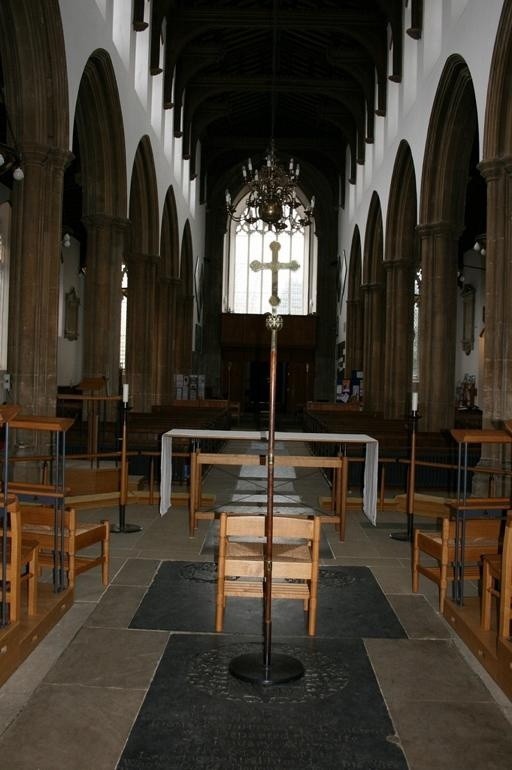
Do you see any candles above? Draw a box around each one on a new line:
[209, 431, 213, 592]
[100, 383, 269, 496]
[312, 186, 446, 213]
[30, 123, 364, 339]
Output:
[412, 392, 418, 411]
[122, 383, 129, 403]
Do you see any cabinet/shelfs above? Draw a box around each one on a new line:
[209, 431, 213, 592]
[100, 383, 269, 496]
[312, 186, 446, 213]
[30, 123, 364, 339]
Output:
[449, 428, 512, 609]
[0, 402, 23, 630]
[0, 416, 75, 593]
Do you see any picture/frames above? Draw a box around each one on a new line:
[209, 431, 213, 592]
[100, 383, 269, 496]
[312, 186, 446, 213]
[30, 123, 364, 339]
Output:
[64, 287, 80, 341]
[195, 324, 201, 353]
[459, 283, 476, 356]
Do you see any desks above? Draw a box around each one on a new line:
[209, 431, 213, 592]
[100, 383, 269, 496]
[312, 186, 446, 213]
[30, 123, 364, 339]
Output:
[159, 428, 380, 545]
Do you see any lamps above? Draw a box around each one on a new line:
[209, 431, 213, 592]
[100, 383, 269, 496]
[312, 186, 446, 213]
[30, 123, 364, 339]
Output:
[473, 233, 486, 255]
[457, 271, 465, 282]
[223, 137, 317, 232]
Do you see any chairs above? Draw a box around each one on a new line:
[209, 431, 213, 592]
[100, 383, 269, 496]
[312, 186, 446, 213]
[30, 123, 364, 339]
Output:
[214, 512, 321, 636]
[0, 492, 40, 626]
[480, 516, 512, 640]
[0, 505, 111, 589]
[410, 517, 507, 616]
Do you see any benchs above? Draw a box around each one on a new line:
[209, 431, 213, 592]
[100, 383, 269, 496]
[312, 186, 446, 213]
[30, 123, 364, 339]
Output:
[56, 406, 230, 480]
[301, 409, 481, 486]
